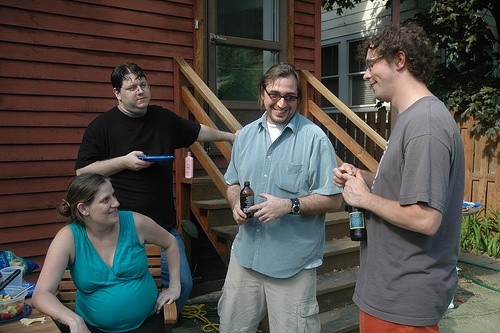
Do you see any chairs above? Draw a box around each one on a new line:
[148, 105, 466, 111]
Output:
[58, 244, 177, 333]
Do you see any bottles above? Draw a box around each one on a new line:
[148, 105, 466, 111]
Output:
[184, 152, 193, 178]
[240, 181, 254, 219]
[347, 205, 368, 242]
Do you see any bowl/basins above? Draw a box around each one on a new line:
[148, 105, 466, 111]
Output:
[0, 286, 28, 320]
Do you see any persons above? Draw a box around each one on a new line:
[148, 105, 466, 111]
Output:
[70, 62, 244, 321]
[30, 171, 182, 333]
[216, 67, 348, 333]
[332, 26, 465, 333]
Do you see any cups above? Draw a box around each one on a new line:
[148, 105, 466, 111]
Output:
[0, 266, 24, 287]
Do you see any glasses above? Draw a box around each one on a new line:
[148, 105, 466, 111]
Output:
[263, 86, 299, 102]
[366, 55, 385, 72]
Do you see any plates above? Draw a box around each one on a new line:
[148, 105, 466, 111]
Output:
[0, 282, 36, 296]
[0, 302, 31, 323]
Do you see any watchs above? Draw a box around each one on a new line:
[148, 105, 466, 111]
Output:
[290, 197, 301, 216]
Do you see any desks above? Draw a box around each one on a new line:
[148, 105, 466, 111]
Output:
[462, 205, 479, 216]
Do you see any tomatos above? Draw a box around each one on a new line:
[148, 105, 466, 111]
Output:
[3, 305, 22, 319]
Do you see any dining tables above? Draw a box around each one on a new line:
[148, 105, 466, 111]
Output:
[0, 279, 61, 333]
